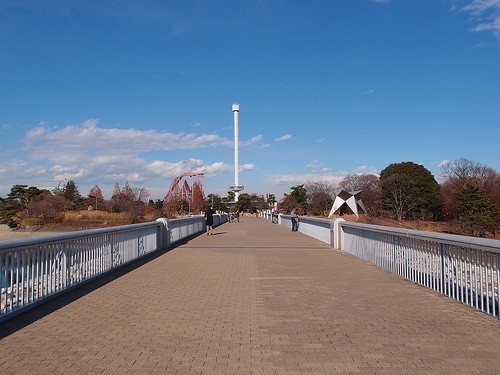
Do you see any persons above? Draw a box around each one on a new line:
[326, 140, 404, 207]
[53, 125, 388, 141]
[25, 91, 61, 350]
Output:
[7, 218, 18, 228]
[290, 208, 298, 232]
[204, 204, 215, 235]
[227, 210, 243, 223]
[251, 209, 258, 218]
